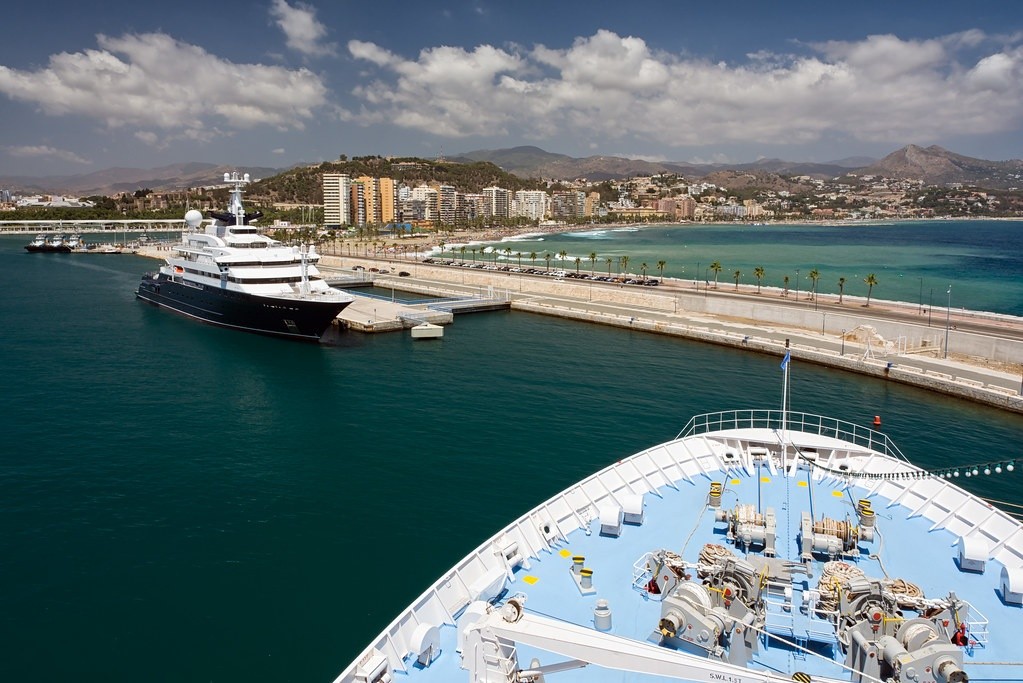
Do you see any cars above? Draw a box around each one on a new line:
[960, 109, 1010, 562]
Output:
[369, 268, 378, 272]
[379, 270, 389, 274]
[352, 265, 364, 270]
[399, 271, 410, 277]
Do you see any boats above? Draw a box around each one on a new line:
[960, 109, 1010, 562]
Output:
[134, 170, 355, 343]
[423, 258, 659, 287]
[23, 225, 50, 252]
[58, 226, 89, 253]
[41, 226, 67, 252]
